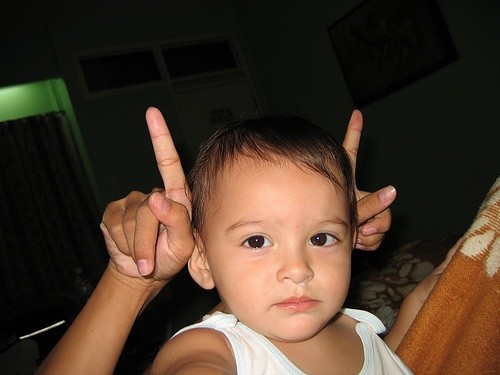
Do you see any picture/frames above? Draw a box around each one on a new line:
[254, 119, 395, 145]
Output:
[328, 0, 459, 108]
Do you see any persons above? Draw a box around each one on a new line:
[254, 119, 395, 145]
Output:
[32, 103, 397, 375]
[150, 113, 464, 375]
